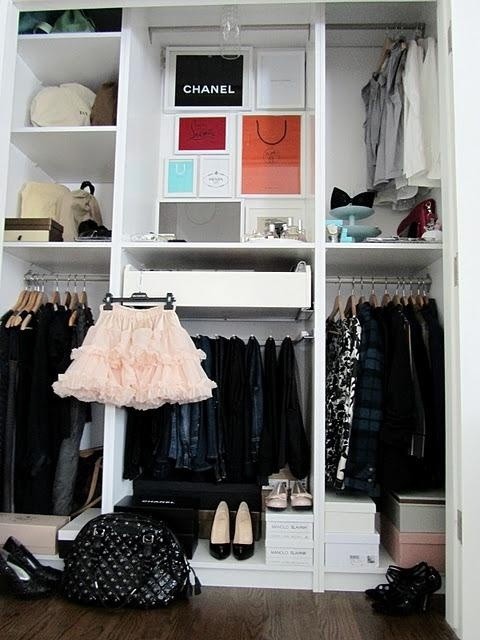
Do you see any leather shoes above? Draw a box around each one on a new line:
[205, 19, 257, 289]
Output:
[210, 500, 231, 560]
[232, 501, 254, 560]
[291, 480, 313, 509]
[0, 536, 64, 601]
[266, 481, 288, 511]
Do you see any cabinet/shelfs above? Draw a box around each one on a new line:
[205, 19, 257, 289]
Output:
[0, 0, 453, 595]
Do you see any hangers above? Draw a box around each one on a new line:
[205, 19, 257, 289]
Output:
[0, 266, 90, 330]
[369, 19, 425, 83]
[327, 274, 430, 336]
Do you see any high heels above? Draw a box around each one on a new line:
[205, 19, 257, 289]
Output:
[365, 562, 441, 611]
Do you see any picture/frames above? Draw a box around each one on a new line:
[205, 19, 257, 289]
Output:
[152, 42, 313, 243]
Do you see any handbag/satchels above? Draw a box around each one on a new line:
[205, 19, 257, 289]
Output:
[65, 511, 202, 609]
[397, 199, 436, 239]
[59, 181, 102, 242]
[72, 446, 102, 510]
[242, 115, 300, 195]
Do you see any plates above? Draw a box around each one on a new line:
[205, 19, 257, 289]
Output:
[338, 225, 382, 243]
[330, 205, 375, 220]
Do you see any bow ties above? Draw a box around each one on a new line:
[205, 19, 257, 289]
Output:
[329, 186, 376, 210]
